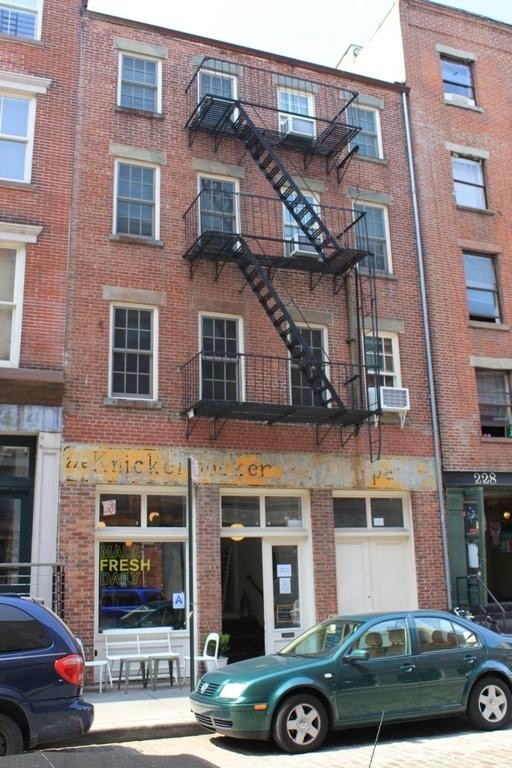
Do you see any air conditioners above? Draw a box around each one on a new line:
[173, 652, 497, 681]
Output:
[288, 234, 322, 259]
[283, 116, 317, 140]
[368, 385, 411, 413]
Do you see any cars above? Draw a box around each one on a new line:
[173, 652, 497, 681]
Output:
[189, 603, 512, 750]
[118, 599, 186, 629]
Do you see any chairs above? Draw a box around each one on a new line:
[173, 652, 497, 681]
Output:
[365, 630, 406, 659]
[419, 630, 465, 653]
[75, 626, 220, 696]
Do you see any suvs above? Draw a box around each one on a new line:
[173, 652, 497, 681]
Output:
[0, 589, 96, 754]
[98, 586, 165, 631]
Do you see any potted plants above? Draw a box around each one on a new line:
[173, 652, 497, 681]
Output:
[201, 631, 230, 673]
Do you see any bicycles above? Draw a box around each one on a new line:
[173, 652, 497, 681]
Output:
[451, 605, 496, 633]
